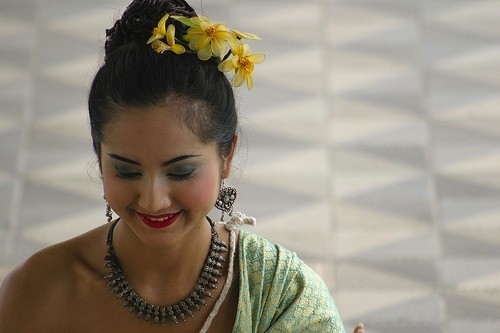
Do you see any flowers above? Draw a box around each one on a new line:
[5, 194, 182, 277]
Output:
[147, 13, 266, 91]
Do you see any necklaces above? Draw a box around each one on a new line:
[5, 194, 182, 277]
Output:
[101, 215, 229, 327]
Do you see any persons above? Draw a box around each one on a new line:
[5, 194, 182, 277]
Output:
[0, 0, 365, 333]
